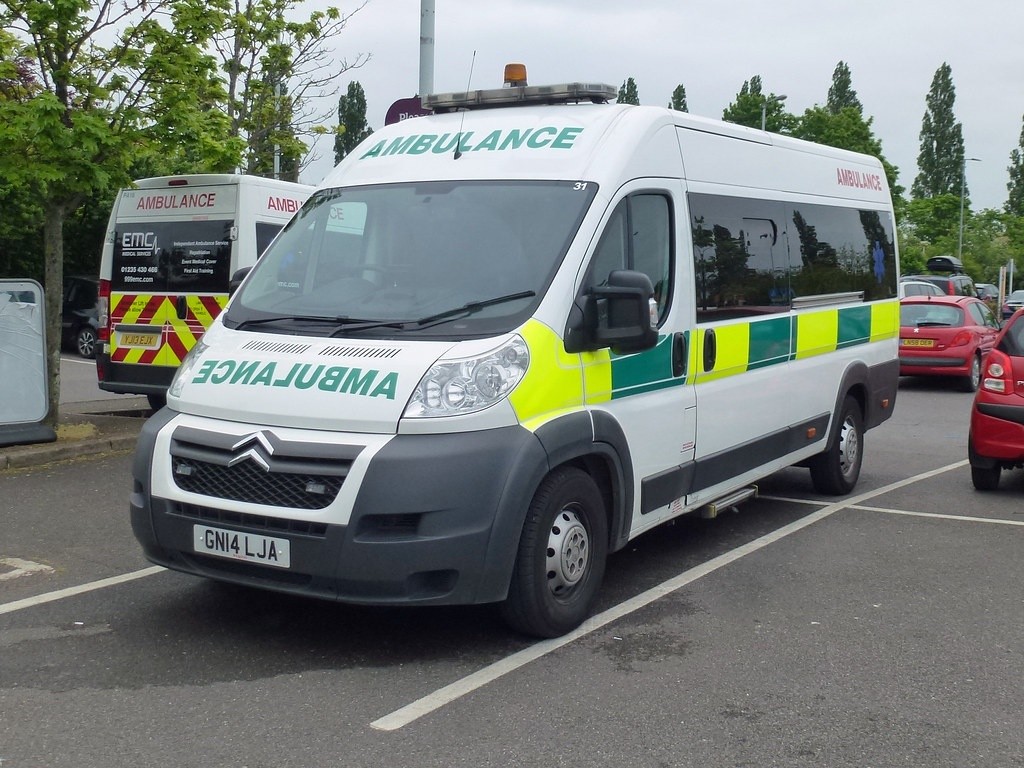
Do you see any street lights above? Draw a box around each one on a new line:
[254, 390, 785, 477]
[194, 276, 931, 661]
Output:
[958, 157, 982, 260]
[759, 94, 788, 131]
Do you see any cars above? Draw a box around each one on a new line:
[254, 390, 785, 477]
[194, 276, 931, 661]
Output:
[974, 282, 999, 300]
[900, 295, 1002, 392]
[967, 307, 1024, 492]
[1004, 290, 1024, 307]
[62, 273, 99, 359]
[899, 281, 946, 299]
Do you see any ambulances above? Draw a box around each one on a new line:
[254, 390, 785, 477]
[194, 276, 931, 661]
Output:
[95, 171, 367, 403]
[127, 64, 899, 638]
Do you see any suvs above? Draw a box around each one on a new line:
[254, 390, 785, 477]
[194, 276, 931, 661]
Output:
[900, 254, 979, 297]
